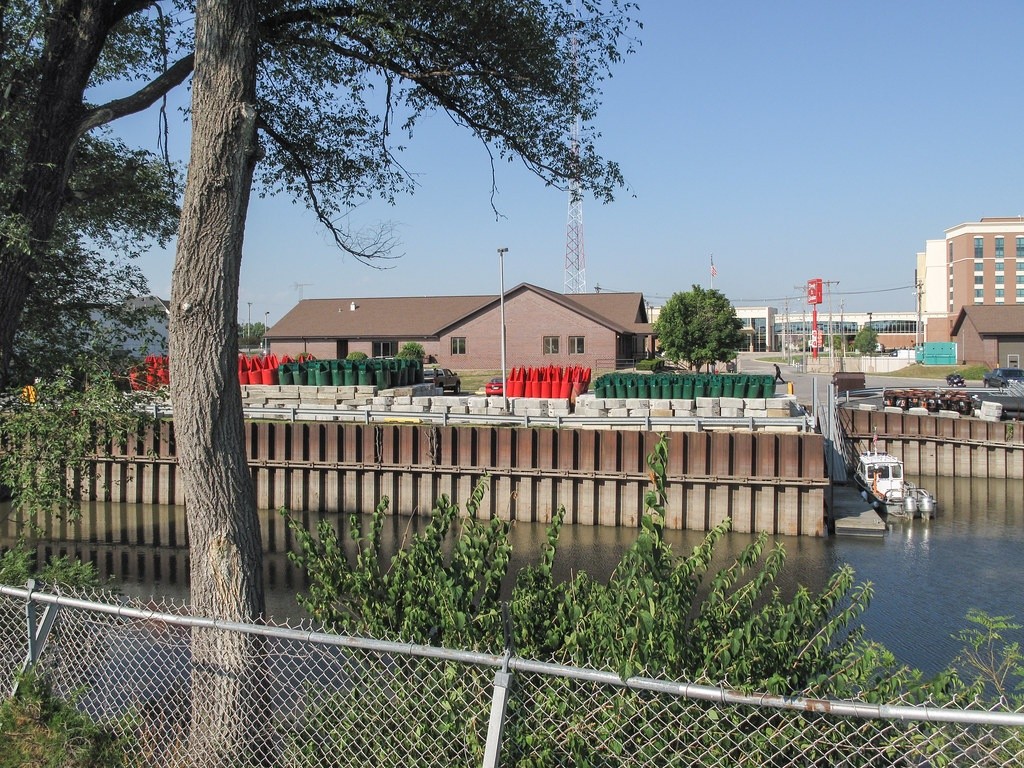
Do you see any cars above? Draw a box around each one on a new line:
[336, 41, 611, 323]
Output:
[889, 350, 898, 357]
[485, 376, 508, 397]
[875, 343, 886, 354]
[983, 367, 1024, 390]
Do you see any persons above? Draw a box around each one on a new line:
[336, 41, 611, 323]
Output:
[774, 364, 785, 384]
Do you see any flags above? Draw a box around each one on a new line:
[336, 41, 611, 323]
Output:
[711, 253, 717, 289]
[873, 427, 878, 455]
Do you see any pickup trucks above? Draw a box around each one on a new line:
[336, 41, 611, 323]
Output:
[423, 368, 461, 394]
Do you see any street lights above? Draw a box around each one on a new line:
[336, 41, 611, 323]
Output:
[248, 303, 253, 344]
[867, 312, 873, 358]
[496, 247, 511, 416]
[264, 312, 270, 354]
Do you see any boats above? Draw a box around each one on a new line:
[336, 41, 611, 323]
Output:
[855, 427, 938, 522]
